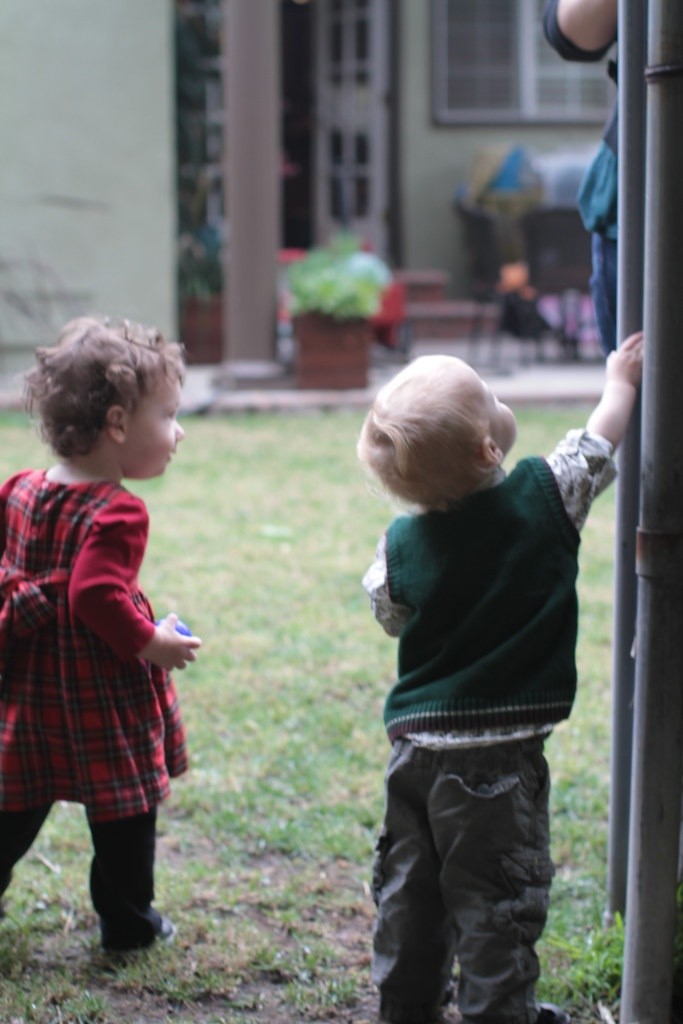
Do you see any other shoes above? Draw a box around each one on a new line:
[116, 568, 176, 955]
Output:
[534, 1004, 570, 1024]
[103, 917, 176, 953]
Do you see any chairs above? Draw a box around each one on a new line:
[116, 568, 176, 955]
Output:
[452, 200, 592, 367]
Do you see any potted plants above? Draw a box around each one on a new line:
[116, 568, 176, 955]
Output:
[282, 235, 394, 390]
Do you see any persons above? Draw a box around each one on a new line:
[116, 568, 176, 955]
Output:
[544, 0, 618, 355]
[355, 328, 645, 1024]
[0, 318, 201, 951]
